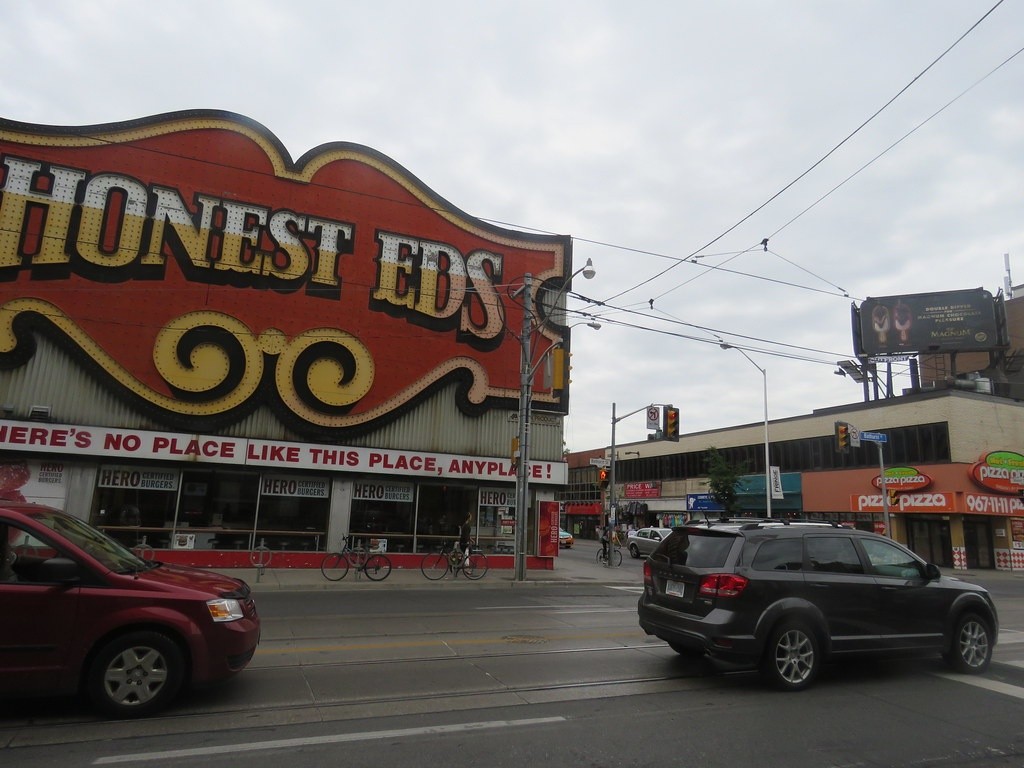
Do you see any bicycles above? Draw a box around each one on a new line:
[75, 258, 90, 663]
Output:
[596, 542, 624, 567]
[420, 537, 489, 581]
[321, 533, 392, 581]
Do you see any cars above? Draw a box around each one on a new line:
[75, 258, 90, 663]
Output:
[626, 528, 673, 559]
[558, 526, 575, 548]
[1, 498, 260, 720]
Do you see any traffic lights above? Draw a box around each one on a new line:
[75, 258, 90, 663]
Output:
[662, 406, 680, 442]
[834, 420, 852, 454]
[599, 469, 609, 485]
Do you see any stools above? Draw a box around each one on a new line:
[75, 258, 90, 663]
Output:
[257, 542, 268, 550]
[159, 538, 170, 549]
[436, 545, 443, 553]
[300, 542, 310, 551]
[208, 539, 219, 548]
[277, 541, 289, 551]
[233, 540, 244, 550]
[396, 544, 404, 552]
[417, 545, 424, 552]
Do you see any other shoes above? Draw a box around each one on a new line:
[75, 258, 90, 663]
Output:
[449, 564, 453, 573]
[603, 560, 607, 563]
[464, 570, 472, 575]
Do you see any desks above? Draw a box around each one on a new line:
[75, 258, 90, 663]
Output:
[94, 525, 516, 555]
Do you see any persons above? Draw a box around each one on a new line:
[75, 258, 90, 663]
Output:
[460, 513, 475, 575]
[0, 539, 19, 582]
[601, 526, 622, 565]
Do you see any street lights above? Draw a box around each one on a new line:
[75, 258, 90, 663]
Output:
[718, 341, 773, 520]
[512, 260, 602, 580]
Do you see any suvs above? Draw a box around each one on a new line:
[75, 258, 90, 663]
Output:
[636, 517, 1000, 693]
